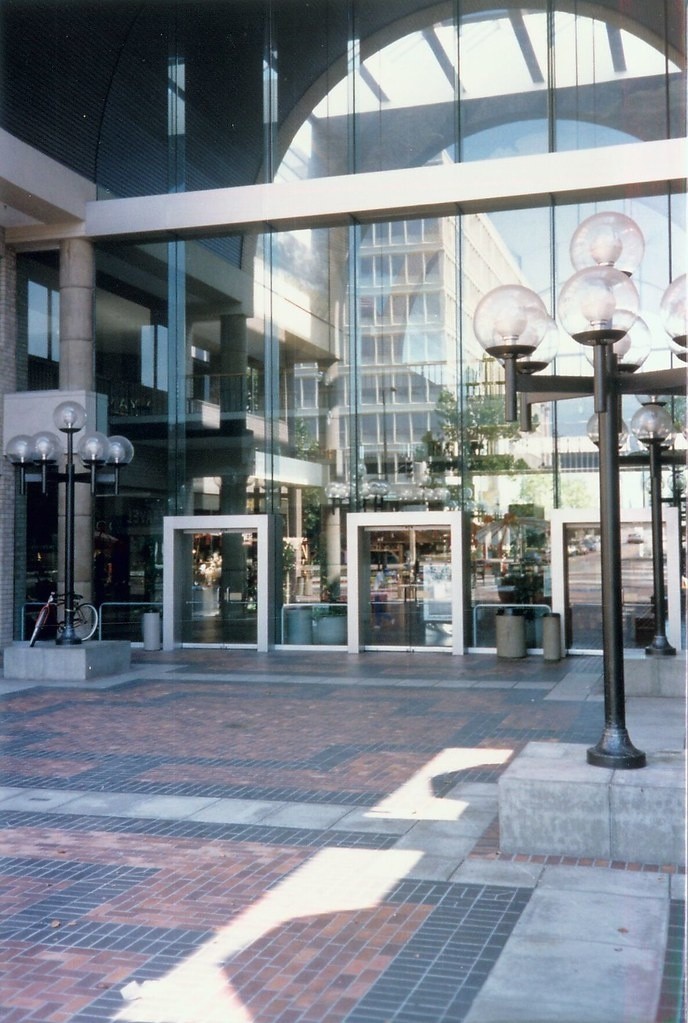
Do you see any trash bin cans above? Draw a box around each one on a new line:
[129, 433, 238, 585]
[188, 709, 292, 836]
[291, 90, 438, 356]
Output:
[143, 608, 162, 652]
[496, 606, 528, 659]
[541, 612, 561, 661]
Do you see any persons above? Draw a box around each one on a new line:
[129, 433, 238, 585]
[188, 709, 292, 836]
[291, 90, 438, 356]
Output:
[500, 552, 507, 576]
[373, 568, 396, 630]
[93, 544, 112, 590]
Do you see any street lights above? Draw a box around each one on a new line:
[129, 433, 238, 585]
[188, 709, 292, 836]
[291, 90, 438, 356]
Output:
[585, 391, 678, 656]
[472, 211, 687, 769]
[6, 400, 136, 647]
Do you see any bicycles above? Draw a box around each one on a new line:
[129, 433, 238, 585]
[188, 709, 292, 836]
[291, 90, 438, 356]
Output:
[28, 577, 99, 646]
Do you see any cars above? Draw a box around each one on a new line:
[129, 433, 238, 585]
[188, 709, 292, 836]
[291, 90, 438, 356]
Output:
[322, 532, 644, 615]
